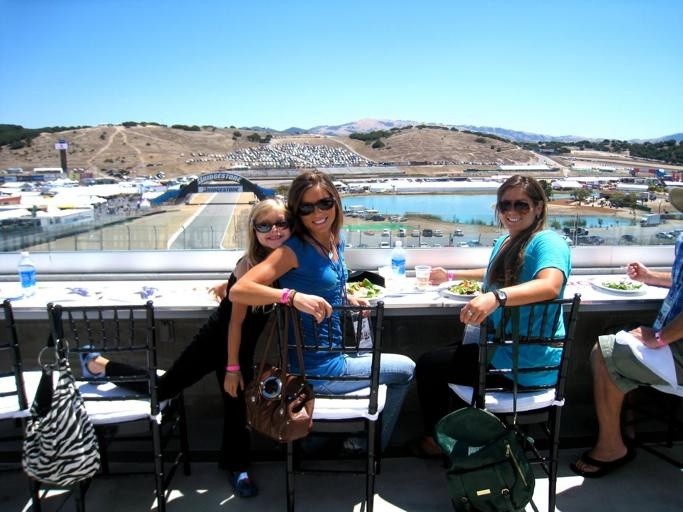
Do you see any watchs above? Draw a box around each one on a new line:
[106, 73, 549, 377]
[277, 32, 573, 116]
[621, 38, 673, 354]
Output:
[492, 289, 507, 306]
[286, 288, 296, 308]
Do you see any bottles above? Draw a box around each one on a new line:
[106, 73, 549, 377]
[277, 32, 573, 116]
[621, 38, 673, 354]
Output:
[18, 252, 36, 297]
[392, 241, 406, 279]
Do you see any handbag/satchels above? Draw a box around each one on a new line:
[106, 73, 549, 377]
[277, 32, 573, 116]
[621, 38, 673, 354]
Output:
[244, 365, 314, 444]
[430, 405, 534, 511]
[20, 359, 101, 487]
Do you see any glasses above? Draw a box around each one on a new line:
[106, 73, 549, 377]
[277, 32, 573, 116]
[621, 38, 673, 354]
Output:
[497, 201, 530, 215]
[253, 220, 289, 233]
[297, 197, 336, 216]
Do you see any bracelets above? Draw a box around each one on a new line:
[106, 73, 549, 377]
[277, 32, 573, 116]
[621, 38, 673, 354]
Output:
[447, 271, 455, 281]
[224, 366, 241, 371]
[281, 287, 289, 304]
[654, 330, 667, 347]
[225, 372, 242, 376]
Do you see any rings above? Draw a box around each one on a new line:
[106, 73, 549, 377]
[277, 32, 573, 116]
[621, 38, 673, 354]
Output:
[467, 309, 474, 318]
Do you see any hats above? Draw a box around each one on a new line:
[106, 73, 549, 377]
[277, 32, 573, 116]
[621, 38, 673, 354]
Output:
[670, 188, 683, 213]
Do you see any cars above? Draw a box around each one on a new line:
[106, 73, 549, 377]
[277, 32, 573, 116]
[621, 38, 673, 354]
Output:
[543, 168, 682, 202]
[562, 213, 683, 245]
[342, 209, 499, 247]
[1, 167, 199, 215]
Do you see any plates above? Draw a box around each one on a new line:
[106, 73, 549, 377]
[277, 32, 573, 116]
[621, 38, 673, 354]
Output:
[589, 275, 648, 294]
[439, 280, 483, 297]
[346, 281, 386, 301]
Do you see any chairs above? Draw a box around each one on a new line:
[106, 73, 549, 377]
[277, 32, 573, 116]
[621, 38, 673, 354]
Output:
[272, 300, 388, 512]
[634, 385, 683, 469]
[448, 293, 580, 509]
[0, 300, 47, 512]
[45, 301, 188, 511]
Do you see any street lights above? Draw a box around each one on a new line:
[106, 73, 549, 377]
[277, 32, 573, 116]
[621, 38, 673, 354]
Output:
[659, 199, 670, 213]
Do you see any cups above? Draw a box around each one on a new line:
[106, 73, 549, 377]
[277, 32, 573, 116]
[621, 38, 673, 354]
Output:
[415, 265, 431, 289]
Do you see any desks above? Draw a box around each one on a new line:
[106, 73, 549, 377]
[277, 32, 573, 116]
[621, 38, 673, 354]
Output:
[0, 274, 670, 322]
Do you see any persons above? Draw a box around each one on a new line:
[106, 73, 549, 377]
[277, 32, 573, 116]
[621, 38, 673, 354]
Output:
[568, 187, 683, 478]
[228, 169, 417, 460]
[78, 197, 292, 497]
[414, 174, 573, 469]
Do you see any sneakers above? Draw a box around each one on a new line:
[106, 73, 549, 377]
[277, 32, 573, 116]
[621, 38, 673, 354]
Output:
[78, 344, 104, 379]
[226, 470, 259, 498]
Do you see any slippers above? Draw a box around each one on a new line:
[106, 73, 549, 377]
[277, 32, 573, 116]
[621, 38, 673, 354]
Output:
[570, 445, 629, 478]
